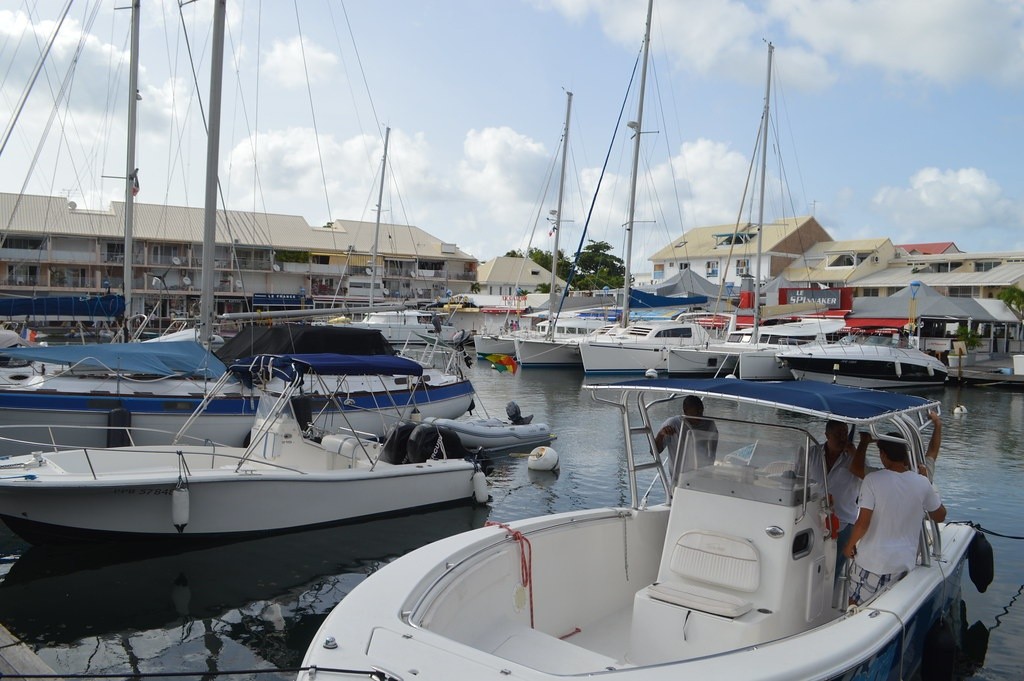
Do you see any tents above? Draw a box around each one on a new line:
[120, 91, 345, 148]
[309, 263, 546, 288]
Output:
[637, 268, 727, 299]
[845, 281, 1018, 355]
[760, 275, 799, 295]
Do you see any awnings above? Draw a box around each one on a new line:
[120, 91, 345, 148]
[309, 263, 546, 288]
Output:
[522, 309, 686, 320]
[696, 316, 755, 327]
[782, 310, 850, 319]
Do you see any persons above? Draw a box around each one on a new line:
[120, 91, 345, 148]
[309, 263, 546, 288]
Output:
[904, 331, 909, 339]
[800, 420, 870, 578]
[650, 395, 719, 498]
[135, 312, 141, 328]
[510, 320, 517, 332]
[40, 340, 47, 348]
[122, 322, 129, 344]
[851, 410, 941, 485]
[981, 327, 985, 336]
[0, 321, 4, 331]
[149, 314, 156, 329]
[17, 316, 37, 349]
[843, 431, 946, 606]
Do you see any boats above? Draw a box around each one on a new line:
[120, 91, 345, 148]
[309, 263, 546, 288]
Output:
[295, 370, 994, 681]
[1, 350, 493, 541]
[395, 403, 555, 454]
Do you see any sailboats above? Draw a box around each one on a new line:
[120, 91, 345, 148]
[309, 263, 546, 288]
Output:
[1, 0, 1024, 458]
